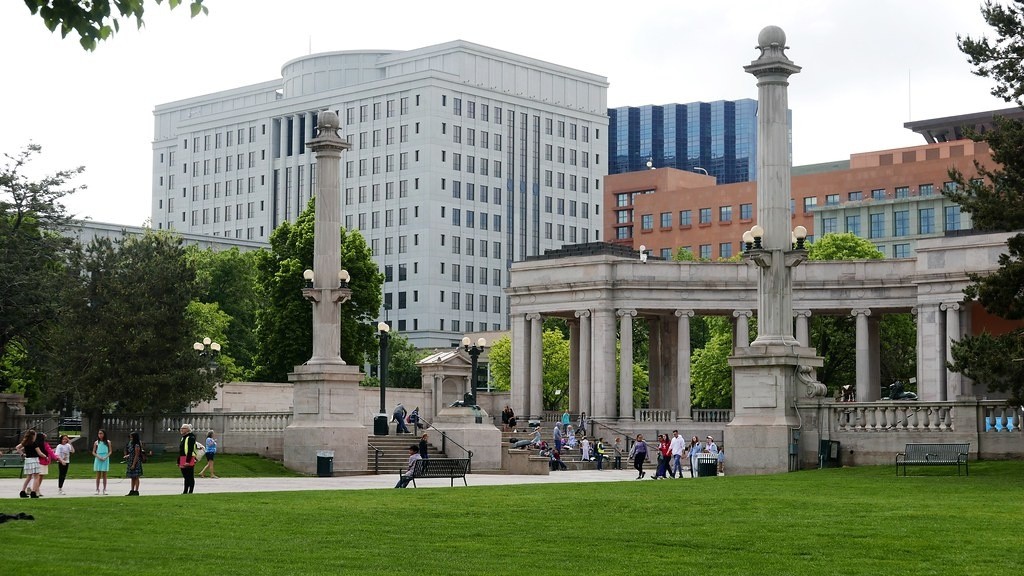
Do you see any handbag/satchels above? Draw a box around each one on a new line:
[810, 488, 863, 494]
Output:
[407, 414, 411, 423]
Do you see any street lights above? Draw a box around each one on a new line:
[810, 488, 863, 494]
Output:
[300, 109, 353, 364]
[374, 322, 391, 414]
[740, 24, 809, 347]
[461, 337, 486, 406]
[193, 337, 222, 382]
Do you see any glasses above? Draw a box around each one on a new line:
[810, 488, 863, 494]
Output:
[181, 428, 188, 430]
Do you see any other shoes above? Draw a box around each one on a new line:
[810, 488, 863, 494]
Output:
[95, 489, 100, 495]
[636, 476, 642, 479]
[651, 472, 683, 479]
[30, 491, 39, 498]
[125, 490, 134, 496]
[132, 491, 139, 496]
[26, 487, 32, 495]
[197, 473, 204, 478]
[35, 490, 43, 496]
[20, 491, 29, 498]
[211, 475, 219, 479]
[103, 489, 108, 495]
[642, 472, 645, 478]
[58, 488, 64, 495]
[404, 430, 411, 433]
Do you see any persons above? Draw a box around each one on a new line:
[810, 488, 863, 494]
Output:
[93, 429, 112, 495]
[15, 429, 75, 498]
[176, 424, 206, 494]
[501, 404, 625, 471]
[390, 403, 424, 434]
[123, 432, 143, 496]
[395, 445, 422, 488]
[419, 434, 429, 459]
[627, 430, 724, 480]
[198, 429, 218, 478]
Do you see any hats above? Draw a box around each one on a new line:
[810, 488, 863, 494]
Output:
[706, 435, 713, 440]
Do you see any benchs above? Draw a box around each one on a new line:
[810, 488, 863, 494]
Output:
[400, 457, 471, 488]
[0, 453, 25, 478]
[142, 444, 180, 463]
[895, 442, 970, 477]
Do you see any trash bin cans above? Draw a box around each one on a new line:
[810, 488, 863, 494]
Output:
[696, 452, 718, 477]
[315, 450, 334, 477]
